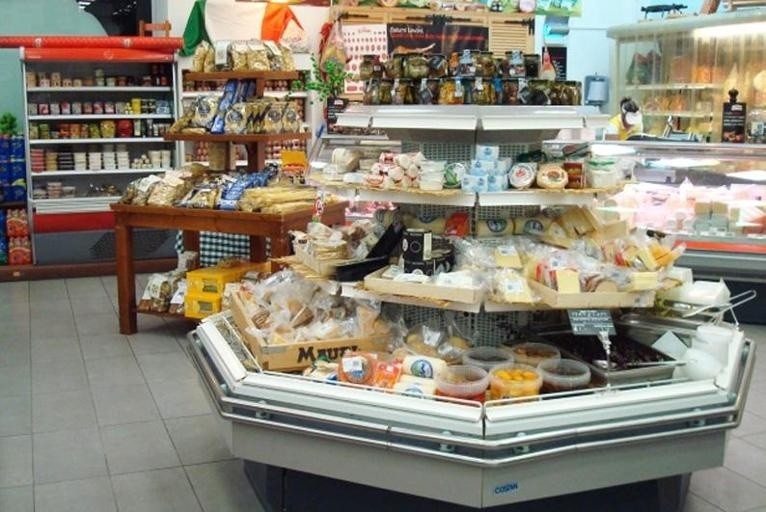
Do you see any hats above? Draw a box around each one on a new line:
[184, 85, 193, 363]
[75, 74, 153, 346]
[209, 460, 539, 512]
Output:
[622, 104, 642, 125]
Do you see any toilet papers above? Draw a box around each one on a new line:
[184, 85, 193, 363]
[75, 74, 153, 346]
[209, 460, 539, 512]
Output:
[589, 81, 607, 102]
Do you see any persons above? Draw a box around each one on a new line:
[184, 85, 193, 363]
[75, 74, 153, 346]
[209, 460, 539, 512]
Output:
[602, 96, 643, 141]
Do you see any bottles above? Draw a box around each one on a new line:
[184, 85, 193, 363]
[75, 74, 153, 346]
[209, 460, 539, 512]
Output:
[0, 209, 32, 266]
[0, 133, 27, 202]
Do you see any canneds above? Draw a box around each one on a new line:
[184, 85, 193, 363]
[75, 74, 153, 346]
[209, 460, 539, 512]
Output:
[359, 49, 583, 106]
